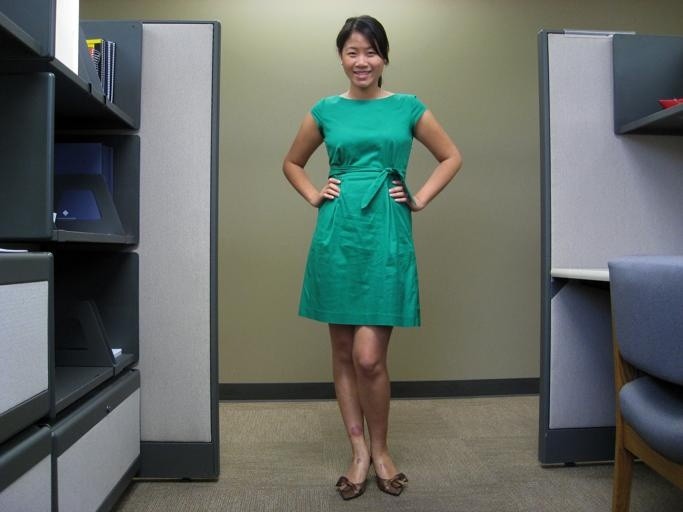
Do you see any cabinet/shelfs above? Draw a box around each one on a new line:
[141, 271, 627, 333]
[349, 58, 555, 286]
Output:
[0, 0, 141, 512]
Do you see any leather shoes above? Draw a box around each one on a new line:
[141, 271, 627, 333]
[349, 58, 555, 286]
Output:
[335, 476, 366, 500]
[375, 473, 408, 497]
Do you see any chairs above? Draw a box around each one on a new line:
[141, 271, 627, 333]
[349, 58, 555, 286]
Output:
[608, 256, 683, 512]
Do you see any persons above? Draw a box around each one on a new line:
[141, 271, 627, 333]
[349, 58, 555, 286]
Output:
[280, 13, 462, 499]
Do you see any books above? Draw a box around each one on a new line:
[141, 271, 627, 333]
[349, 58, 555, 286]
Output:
[85, 37, 115, 103]
